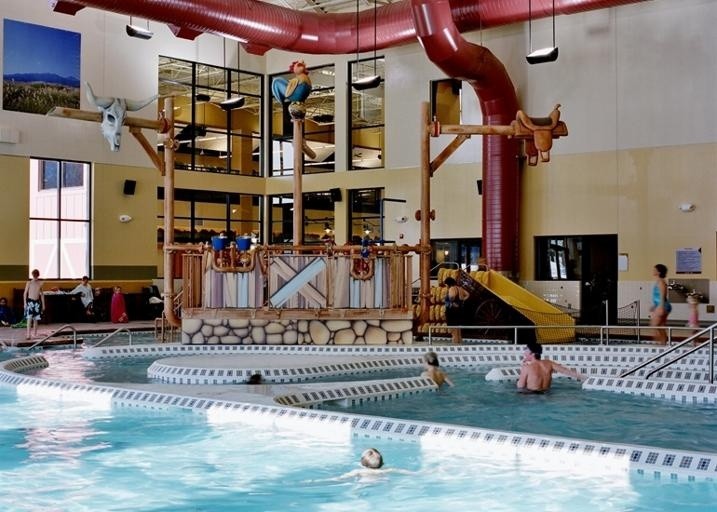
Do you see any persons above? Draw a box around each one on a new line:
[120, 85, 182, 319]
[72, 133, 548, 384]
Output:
[422, 353, 454, 390]
[42, 286, 63, 297]
[0, 297, 13, 327]
[21, 270, 44, 340]
[687, 290, 697, 342]
[444, 276, 467, 343]
[518, 342, 588, 392]
[68, 276, 102, 315]
[112, 287, 128, 323]
[647, 264, 670, 342]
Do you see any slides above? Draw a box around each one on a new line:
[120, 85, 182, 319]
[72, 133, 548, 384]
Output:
[470, 269, 577, 345]
